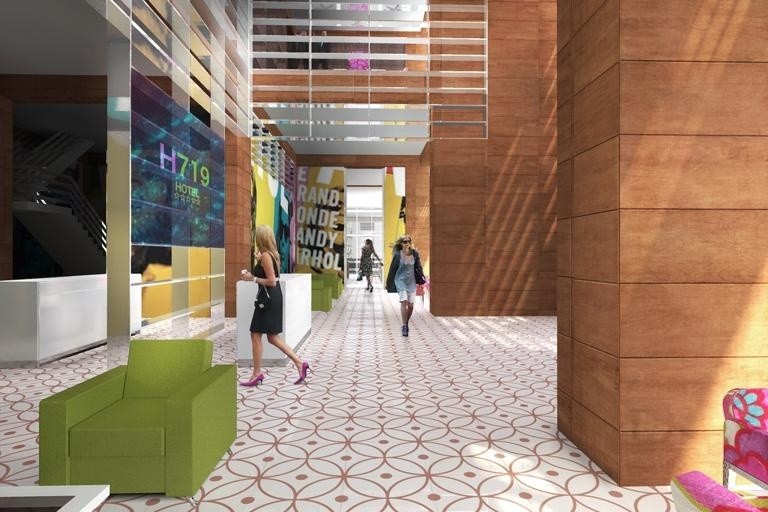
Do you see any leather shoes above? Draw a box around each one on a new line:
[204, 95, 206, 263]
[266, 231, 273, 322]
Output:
[403, 325, 408, 336]
[366, 285, 374, 293]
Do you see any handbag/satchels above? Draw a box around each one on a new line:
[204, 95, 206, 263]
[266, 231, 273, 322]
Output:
[253, 285, 272, 313]
[357, 272, 363, 281]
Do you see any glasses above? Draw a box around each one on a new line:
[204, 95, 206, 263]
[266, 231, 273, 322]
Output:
[402, 240, 411, 243]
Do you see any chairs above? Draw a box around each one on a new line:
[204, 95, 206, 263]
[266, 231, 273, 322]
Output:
[35, 338, 239, 504]
[300, 264, 344, 312]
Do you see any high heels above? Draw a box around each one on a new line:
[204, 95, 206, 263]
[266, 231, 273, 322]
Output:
[293, 362, 313, 385]
[240, 373, 264, 387]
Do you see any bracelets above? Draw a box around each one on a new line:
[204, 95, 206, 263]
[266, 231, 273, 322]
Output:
[253, 277, 257, 283]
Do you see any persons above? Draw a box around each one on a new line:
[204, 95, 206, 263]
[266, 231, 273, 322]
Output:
[385, 235, 425, 337]
[239, 225, 316, 385]
[296, 31, 310, 68]
[311, 30, 331, 69]
[358, 239, 383, 292]
